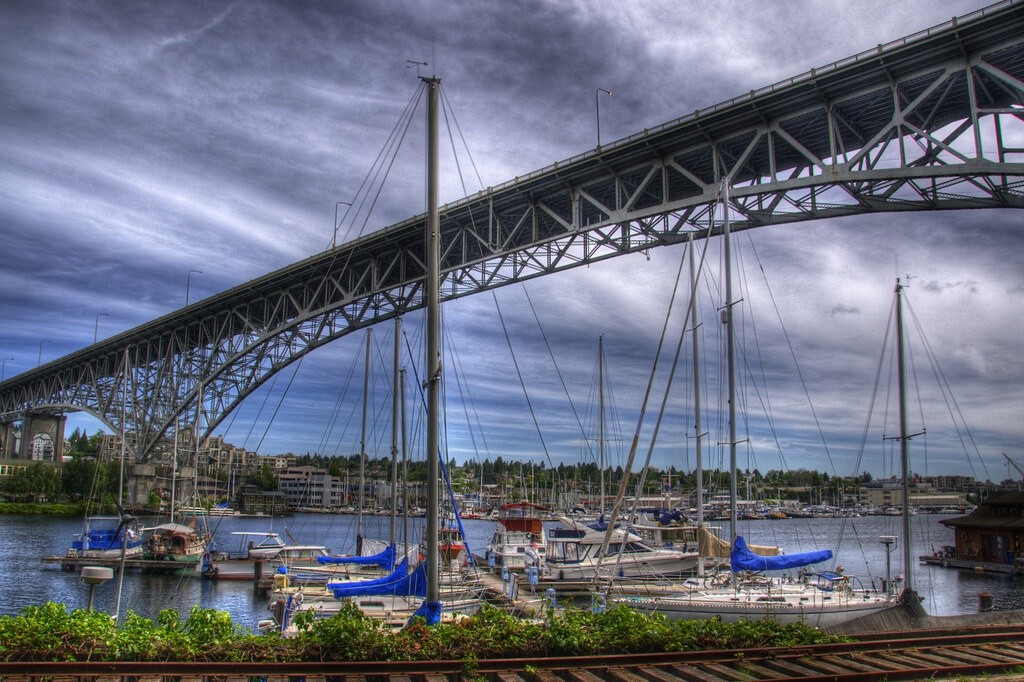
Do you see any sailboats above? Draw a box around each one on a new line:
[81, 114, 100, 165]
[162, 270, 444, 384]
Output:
[0, 175, 995, 635]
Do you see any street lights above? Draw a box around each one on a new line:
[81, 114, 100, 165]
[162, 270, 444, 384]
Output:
[185, 270, 203, 307]
[1, 357, 14, 381]
[596, 87, 614, 145]
[94, 313, 110, 343]
[38, 340, 52, 365]
[333, 202, 353, 247]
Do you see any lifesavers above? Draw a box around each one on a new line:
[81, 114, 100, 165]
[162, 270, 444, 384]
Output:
[291, 593, 304, 605]
[526, 532, 533, 539]
[960, 531, 969, 542]
